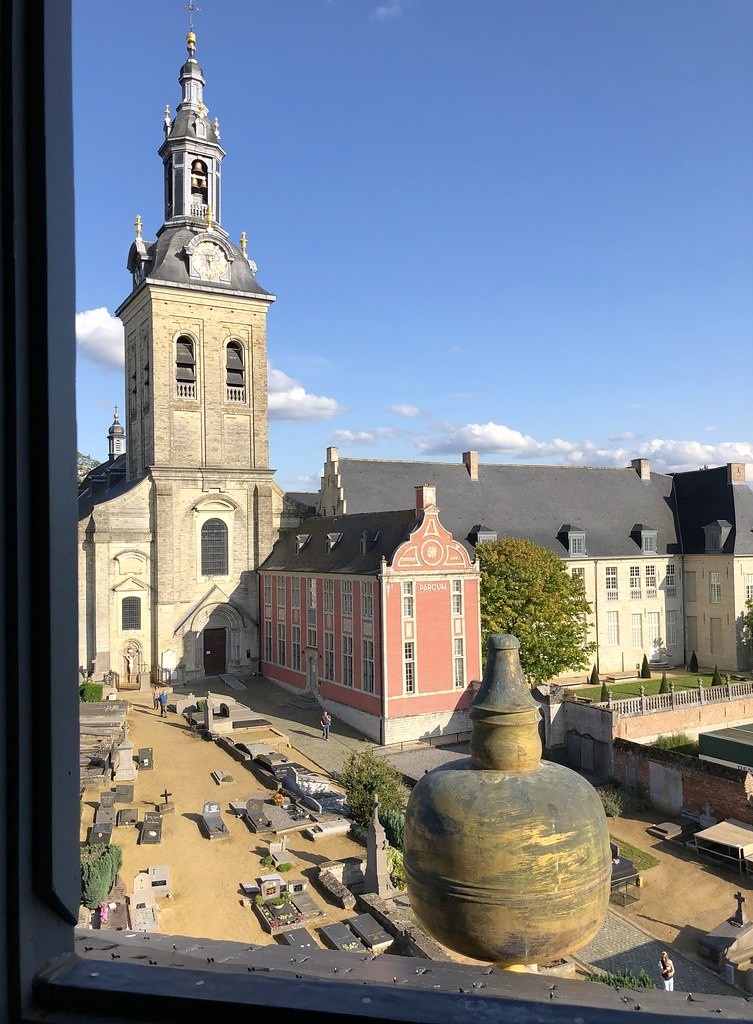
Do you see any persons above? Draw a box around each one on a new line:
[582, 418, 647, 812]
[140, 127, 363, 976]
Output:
[658, 952, 675, 991]
[126, 653, 138, 683]
[153, 686, 160, 710]
[321, 710, 331, 741]
[159, 690, 167, 718]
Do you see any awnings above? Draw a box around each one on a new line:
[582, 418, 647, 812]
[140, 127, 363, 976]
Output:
[227, 348, 245, 371]
[176, 368, 196, 381]
[227, 373, 246, 386]
[144, 376, 149, 385]
[144, 361, 149, 370]
[132, 371, 136, 378]
[132, 386, 137, 393]
[176, 344, 195, 364]
